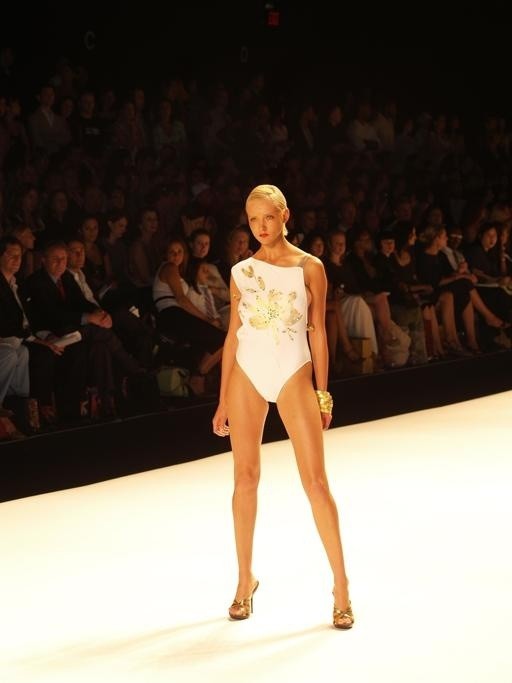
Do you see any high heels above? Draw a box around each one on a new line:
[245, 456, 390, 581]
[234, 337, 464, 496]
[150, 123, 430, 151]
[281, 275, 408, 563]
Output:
[486, 319, 511, 332]
[187, 371, 218, 402]
[467, 345, 483, 355]
[443, 338, 463, 355]
[332, 590, 354, 629]
[228, 581, 260, 619]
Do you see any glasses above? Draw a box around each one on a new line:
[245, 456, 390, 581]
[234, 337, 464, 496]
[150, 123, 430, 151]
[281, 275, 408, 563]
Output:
[450, 233, 464, 240]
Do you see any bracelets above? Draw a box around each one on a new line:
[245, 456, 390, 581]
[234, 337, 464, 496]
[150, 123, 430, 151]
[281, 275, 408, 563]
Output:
[315, 390, 333, 415]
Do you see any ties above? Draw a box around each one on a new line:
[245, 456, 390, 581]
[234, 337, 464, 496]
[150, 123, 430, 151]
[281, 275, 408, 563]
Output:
[55, 278, 64, 297]
[453, 250, 460, 267]
[203, 287, 214, 321]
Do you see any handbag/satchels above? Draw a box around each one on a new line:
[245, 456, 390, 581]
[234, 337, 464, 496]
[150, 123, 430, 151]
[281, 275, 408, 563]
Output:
[340, 336, 374, 376]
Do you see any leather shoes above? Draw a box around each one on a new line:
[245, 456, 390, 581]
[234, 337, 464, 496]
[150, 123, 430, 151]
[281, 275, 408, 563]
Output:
[103, 391, 118, 421]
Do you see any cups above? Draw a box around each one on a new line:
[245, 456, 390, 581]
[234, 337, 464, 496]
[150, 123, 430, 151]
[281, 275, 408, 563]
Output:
[88, 392, 98, 420]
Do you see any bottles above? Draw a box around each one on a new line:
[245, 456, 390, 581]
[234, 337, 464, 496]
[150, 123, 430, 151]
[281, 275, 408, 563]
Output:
[334, 284, 345, 301]
[28, 399, 40, 429]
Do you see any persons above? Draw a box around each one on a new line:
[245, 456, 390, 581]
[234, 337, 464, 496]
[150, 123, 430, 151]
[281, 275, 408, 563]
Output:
[1, 0, 511, 440]
[210, 181, 355, 632]
